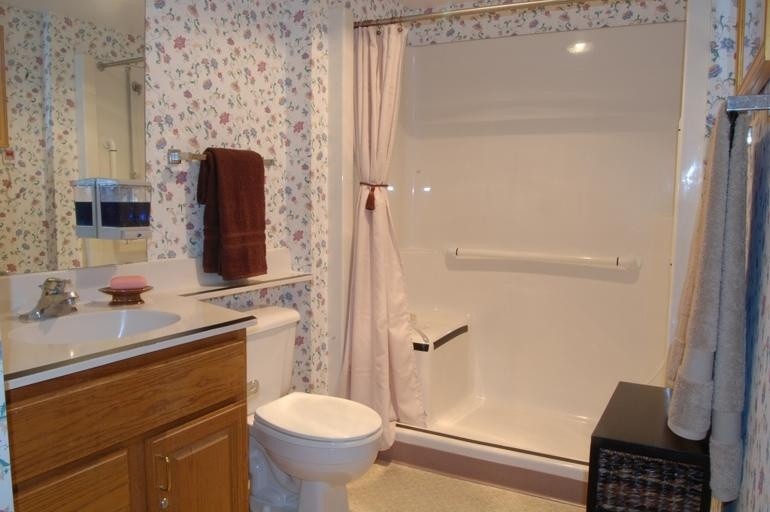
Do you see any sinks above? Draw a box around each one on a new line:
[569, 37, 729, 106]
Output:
[7, 310, 181, 345]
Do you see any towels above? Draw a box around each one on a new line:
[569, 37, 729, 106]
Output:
[195, 147, 268, 281]
[663, 100, 746, 505]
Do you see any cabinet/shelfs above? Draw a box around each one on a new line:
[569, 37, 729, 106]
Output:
[7, 326, 247, 512]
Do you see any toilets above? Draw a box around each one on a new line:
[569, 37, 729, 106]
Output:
[239, 307, 384, 510]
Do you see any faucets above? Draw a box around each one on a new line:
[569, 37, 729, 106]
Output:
[20, 277, 80, 323]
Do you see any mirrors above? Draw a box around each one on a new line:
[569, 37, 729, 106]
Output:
[1, 0, 147, 274]
